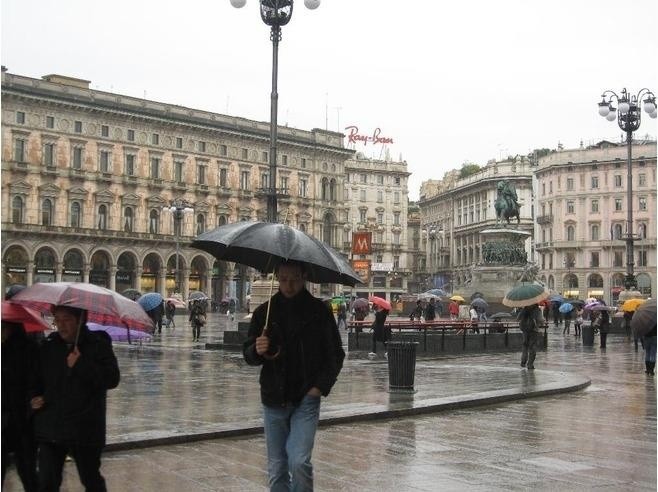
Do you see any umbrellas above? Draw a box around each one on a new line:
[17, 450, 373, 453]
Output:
[7, 282, 157, 353]
[191, 220, 364, 337]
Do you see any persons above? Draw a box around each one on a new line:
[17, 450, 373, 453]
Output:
[242, 259, 348, 491]
[336, 298, 657, 375]
[2, 317, 47, 492]
[34, 303, 120, 491]
[2, 281, 239, 344]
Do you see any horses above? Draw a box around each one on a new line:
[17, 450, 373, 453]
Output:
[493, 180, 522, 227]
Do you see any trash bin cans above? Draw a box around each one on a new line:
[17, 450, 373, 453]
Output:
[384, 341, 419, 391]
[582, 324, 594, 346]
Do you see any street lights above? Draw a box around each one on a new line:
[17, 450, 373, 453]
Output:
[422, 223, 444, 289]
[163, 202, 196, 301]
[231, 0, 320, 316]
[597, 88, 656, 326]
[562, 254, 576, 298]
